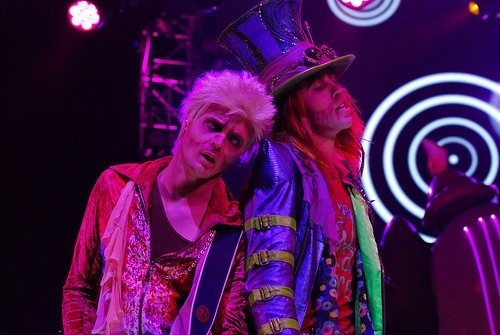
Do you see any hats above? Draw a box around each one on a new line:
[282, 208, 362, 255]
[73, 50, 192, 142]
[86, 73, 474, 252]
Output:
[258, 39, 357, 101]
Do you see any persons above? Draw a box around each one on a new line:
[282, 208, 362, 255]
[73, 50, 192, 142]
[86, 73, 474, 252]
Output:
[217, 0, 386, 335]
[62, 71, 277, 335]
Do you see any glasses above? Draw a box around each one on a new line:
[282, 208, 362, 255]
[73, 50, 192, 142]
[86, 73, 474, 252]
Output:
[294, 45, 338, 65]
